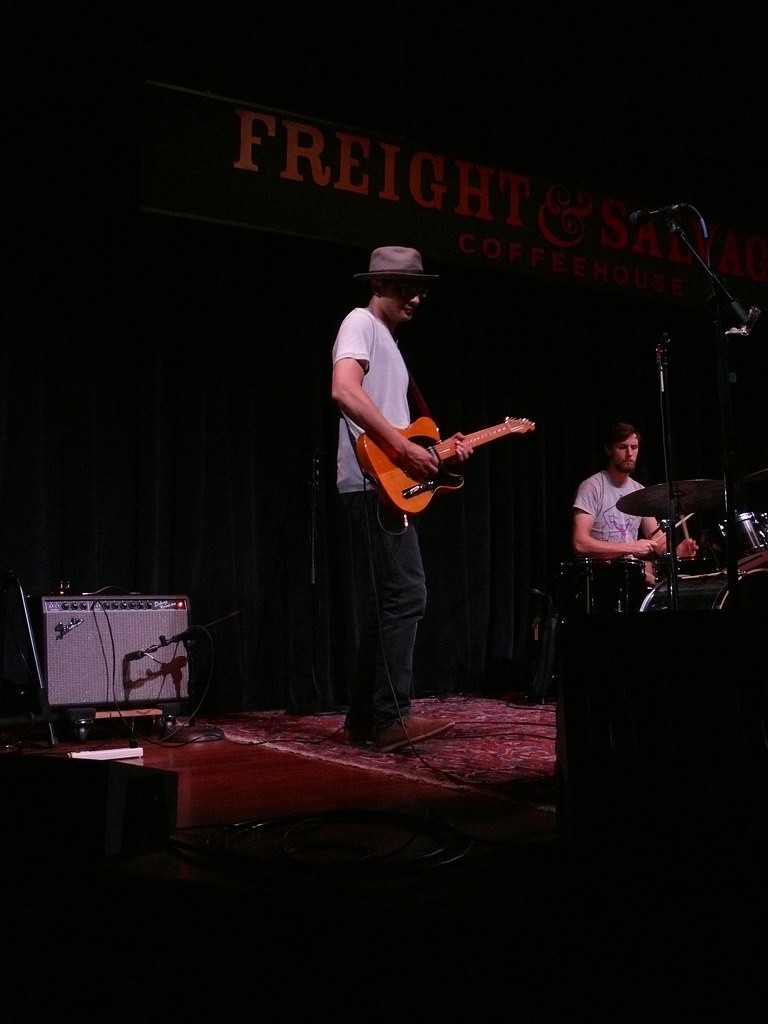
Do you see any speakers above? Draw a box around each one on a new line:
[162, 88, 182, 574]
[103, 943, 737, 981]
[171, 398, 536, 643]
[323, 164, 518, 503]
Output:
[551, 609, 768, 878]
[40, 592, 193, 723]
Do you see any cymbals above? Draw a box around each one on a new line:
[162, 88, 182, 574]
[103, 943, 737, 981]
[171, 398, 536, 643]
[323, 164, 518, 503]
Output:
[615, 477, 725, 517]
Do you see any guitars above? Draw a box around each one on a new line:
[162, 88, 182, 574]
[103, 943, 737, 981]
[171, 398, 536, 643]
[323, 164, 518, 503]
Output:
[356, 414, 538, 516]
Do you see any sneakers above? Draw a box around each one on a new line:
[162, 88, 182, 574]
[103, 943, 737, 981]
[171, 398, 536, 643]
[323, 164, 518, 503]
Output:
[374, 713, 456, 754]
[343, 726, 367, 745]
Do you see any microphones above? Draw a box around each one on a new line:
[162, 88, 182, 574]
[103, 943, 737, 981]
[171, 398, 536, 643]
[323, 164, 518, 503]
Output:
[658, 340, 665, 393]
[630, 203, 690, 227]
[124, 651, 146, 662]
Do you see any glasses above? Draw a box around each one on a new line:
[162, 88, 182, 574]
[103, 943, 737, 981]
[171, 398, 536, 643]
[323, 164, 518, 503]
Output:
[381, 284, 429, 298]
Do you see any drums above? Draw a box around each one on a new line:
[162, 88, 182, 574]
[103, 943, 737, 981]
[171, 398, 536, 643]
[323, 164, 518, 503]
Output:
[554, 554, 654, 615]
[639, 569, 768, 610]
[701, 508, 768, 571]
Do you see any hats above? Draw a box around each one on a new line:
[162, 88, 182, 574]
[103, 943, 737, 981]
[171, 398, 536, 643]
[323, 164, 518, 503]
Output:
[348, 244, 440, 288]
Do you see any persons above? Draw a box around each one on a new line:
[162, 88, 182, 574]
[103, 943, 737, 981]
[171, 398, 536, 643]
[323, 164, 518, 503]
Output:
[572, 423, 700, 560]
[331, 249, 474, 756]
